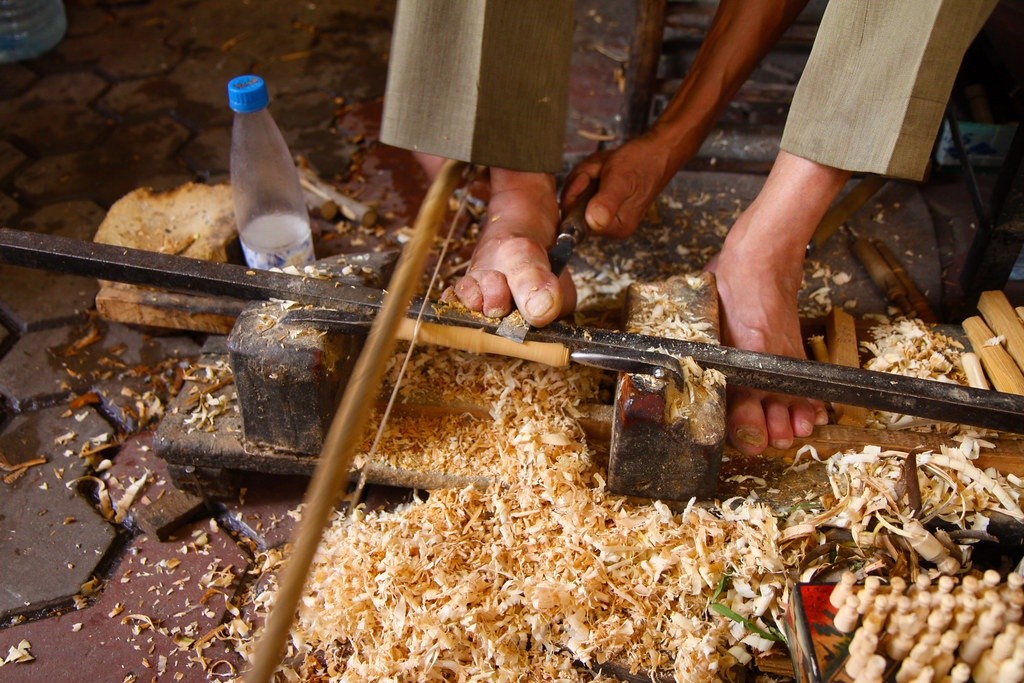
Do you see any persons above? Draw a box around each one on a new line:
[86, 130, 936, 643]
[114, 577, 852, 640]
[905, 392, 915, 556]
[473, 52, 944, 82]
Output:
[378, 1, 994, 455]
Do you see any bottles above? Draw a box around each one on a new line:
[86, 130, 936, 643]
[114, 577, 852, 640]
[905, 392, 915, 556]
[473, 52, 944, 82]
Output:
[0, 0, 67, 61]
[226, 75, 317, 272]
